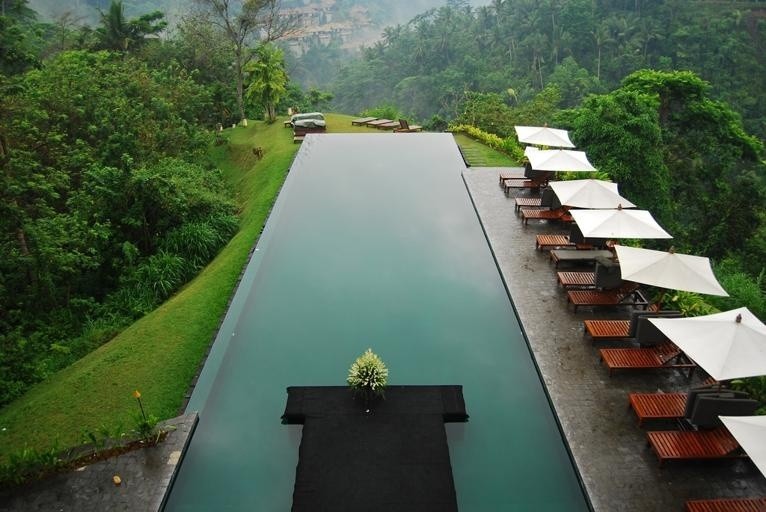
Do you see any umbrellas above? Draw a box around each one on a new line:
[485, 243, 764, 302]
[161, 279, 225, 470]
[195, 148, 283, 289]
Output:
[614, 245, 730, 314]
[515, 125, 673, 245]
[646, 306, 766, 397]
[717, 415, 766, 479]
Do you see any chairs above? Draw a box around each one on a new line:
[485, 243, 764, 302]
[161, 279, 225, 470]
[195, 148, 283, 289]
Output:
[513, 196, 576, 225]
[500, 171, 564, 194]
[535, 233, 622, 269]
[583, 310, 699, 379]
[556, 270, 652, 313]
[630, 377, 766, 512]
[350, 116, 425, 134]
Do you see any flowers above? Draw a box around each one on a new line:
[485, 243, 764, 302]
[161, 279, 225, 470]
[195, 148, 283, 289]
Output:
[345, 348, 389, 402]
